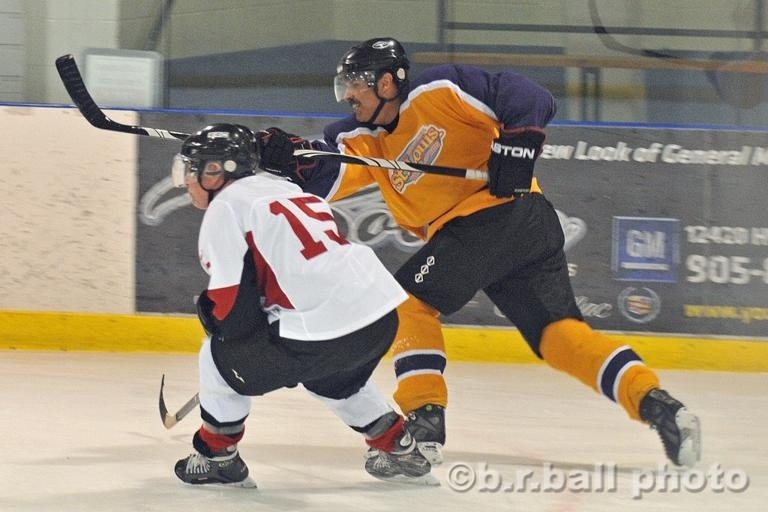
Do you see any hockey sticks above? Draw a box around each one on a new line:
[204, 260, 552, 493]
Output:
[159, 375, 200, 429]
[55, 53, 489, 181]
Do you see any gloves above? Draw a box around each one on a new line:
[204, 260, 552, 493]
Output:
[486, 130, 545, 198]
[252, 125, 318, 188]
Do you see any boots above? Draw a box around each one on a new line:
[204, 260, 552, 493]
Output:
[402, 405, 446, 447]
[174, 443, 249, 485]
[364, 427, 432, 480]
[638, 387, 702, 467]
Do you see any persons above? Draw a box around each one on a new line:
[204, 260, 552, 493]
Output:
[174, 123, 430, 485]
[256, 38, 701, 468]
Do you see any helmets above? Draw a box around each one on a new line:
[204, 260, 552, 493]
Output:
[180, 123, 262, 174]
[336, 36, 408, 110]
